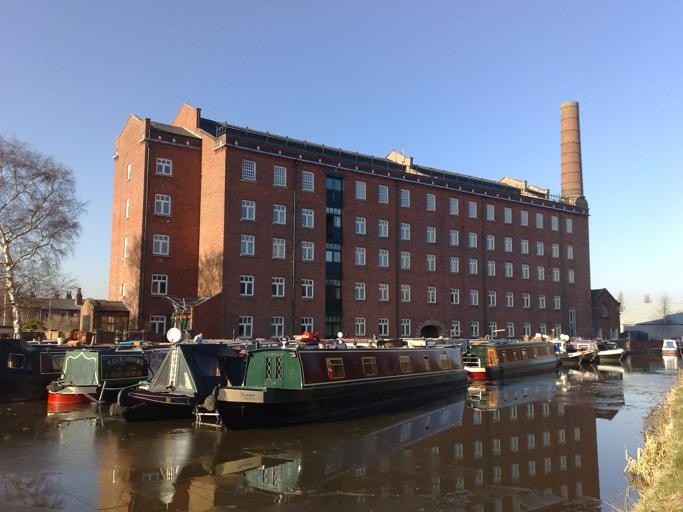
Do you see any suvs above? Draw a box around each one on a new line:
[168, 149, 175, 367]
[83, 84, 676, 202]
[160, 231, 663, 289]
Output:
[616, 330, 648, 339]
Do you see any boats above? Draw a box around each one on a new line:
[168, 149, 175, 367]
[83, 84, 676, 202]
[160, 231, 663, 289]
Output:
[215, 346, 469, 432]
[0, 340, 245, 418]
[661, 337, 682, 357]
[462, 334, 626, 380]
